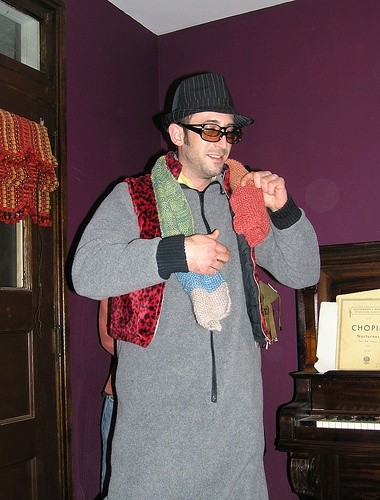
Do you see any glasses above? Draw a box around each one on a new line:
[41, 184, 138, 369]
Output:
[178, 123, 242, 144]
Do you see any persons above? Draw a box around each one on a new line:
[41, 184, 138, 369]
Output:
[70, 73, 323, 500]
[98, 296, 113, 500]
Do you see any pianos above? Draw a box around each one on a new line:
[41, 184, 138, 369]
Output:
[274, 241, 380, 500]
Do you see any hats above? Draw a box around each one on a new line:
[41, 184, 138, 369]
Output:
[161, 72, 255, 130]
